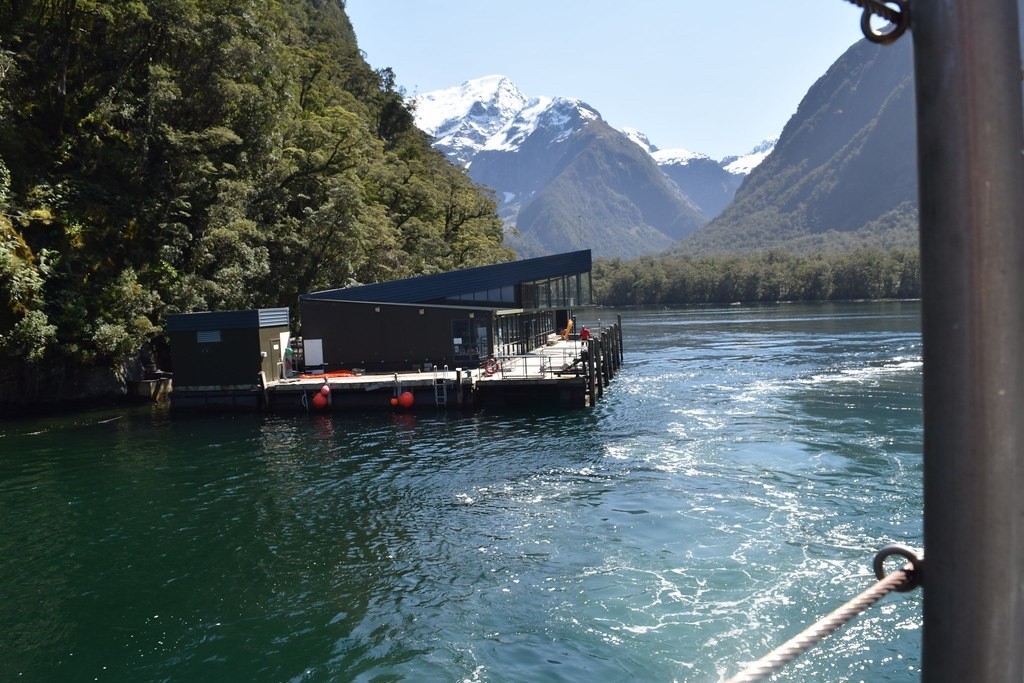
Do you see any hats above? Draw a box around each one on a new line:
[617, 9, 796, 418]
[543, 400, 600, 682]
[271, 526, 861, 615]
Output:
[582, 325, 584, 328]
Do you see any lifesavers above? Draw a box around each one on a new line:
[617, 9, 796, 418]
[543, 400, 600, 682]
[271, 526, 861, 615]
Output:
[484, 358, 499, 374]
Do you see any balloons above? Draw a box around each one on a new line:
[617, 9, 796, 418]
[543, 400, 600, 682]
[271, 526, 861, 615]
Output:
[287, 348, 293, 356]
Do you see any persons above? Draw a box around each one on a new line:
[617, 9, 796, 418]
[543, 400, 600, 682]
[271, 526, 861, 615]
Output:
[579, 325, 591, 349]
